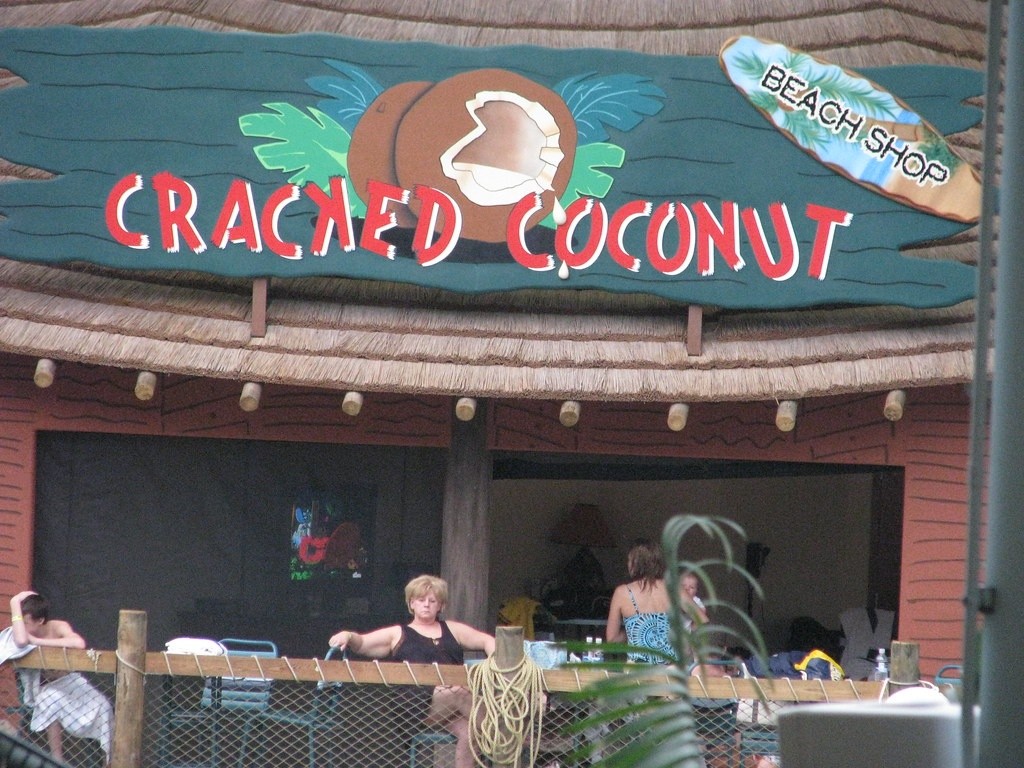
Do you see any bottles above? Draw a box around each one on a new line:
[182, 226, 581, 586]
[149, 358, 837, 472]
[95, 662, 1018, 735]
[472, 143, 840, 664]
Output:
[874, 648, 888, 681]
[582, 636, 605, 663]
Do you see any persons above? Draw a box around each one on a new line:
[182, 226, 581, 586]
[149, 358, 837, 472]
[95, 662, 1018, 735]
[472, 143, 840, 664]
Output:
[606, 536, 709, 675]
[329, 575, 531, 768]
[9, 590, 112, 763]
[688, 622, 742, 768]
[726, 656, 746, 678]
[678, 569, 707, 665]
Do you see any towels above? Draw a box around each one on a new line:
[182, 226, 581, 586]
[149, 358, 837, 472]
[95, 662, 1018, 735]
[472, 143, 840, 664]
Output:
[164, 637, 228, 657]
[0, 627, 115, 765]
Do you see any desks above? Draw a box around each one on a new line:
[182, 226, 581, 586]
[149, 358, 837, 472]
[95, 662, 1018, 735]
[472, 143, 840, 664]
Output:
[554, 618, 624, 659]
[163, 675, 224, 767]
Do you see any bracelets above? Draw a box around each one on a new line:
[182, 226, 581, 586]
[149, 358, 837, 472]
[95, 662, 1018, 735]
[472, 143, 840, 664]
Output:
[11, 615, 24, 622]
[349, 634, 352, 639]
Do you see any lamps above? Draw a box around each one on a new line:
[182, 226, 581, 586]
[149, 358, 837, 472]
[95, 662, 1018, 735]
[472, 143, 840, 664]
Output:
[550, 504, 618, 591]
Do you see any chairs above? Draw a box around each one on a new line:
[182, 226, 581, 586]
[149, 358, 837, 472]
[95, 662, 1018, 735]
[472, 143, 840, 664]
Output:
[409, 731, 457, 768]
[741, 721, 779, 768]
[231, 645, 346, 768]
[12, 671, 33, 737]
[535, 692, 587, 768]
[686, 660, 747, 768]
[174, 640, 277, 766]
